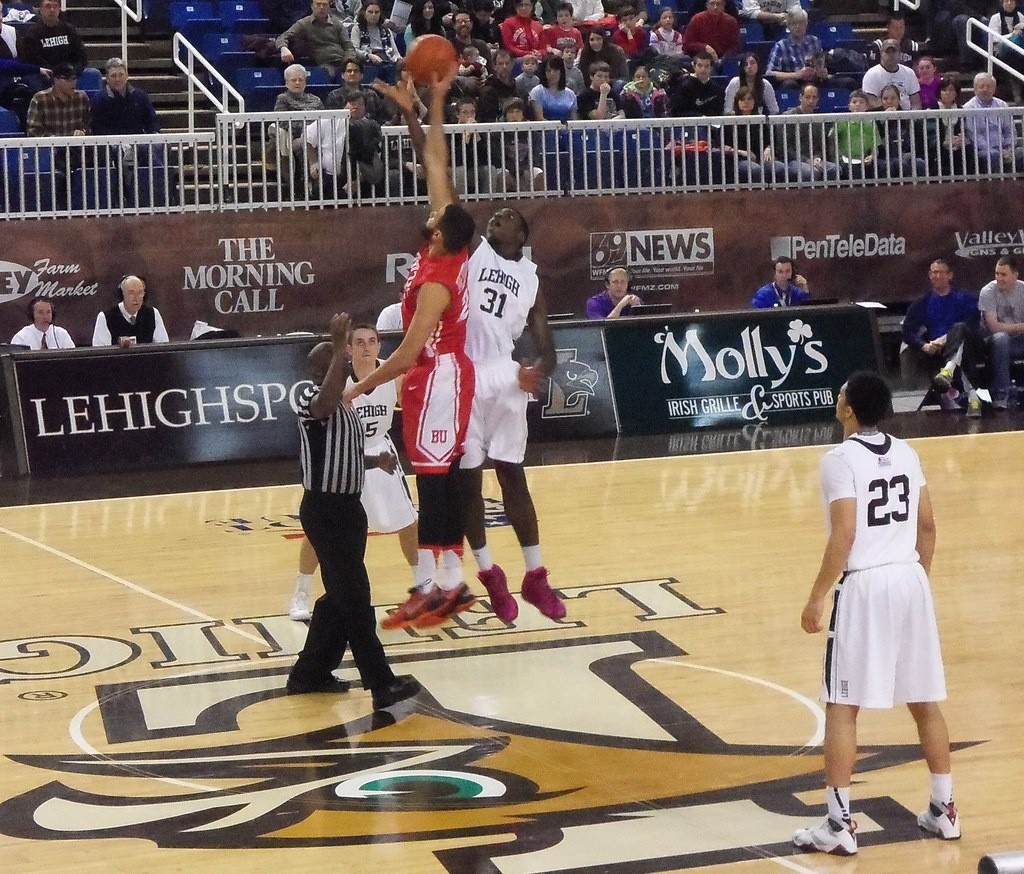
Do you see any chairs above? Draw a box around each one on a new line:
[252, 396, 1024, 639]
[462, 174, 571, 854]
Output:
[0, 0, 870, 210]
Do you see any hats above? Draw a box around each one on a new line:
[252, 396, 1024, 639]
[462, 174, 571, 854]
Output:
[882, 39, 900, 52]
[52, 63, 82, 78]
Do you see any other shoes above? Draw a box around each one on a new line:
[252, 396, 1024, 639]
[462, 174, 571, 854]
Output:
[992, 399, 1008, 409]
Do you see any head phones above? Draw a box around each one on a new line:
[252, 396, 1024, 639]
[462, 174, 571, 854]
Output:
[605, 266, 631, 291]
[770, 259, 800, 278]
[118, 275, 147, 302]
[27, 296, 57, 320]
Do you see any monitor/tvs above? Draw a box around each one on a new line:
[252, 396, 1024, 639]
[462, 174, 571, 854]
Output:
[631, 304, 672, 315]
[800, 297, 838, 306]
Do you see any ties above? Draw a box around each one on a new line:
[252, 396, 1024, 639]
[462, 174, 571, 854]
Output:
[130, 316, 135, 325]
[42, 333, 48, 348]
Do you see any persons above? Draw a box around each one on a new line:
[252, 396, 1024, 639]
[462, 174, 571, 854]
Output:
[286, 313, 422, 711]
[611, 0, 1024, 186]
[750, 255, 811, 308]
[794, 368, 962, 856]
[0, 0, 172, 217]
[11, 295, 76, 350]
[290, 322, 418, 621]
[979, 255, 1024, 410]
[92, 274, 169, 346]
[587, 265, 644, 319]
[899, 258, 988, 417]
[342, 59, 566, 630]
[274, 0, 649, 198]
[376, 286, 403, 330]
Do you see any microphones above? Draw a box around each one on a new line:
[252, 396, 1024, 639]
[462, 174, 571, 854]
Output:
[787, 279, 792, 282]
[50, 322, 53, 324]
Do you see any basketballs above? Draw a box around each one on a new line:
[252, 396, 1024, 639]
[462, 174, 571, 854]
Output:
[407, 34, 456, 86]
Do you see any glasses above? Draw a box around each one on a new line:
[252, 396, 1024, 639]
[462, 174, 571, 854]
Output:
[58, 76, 79, 82]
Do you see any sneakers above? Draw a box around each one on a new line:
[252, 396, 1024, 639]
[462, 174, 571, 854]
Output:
[415, 581, 476, 628]
[286, 675, 351, 695]
[917, 811, 961, 839]
[381, 578, 450, 629]
[477, 564, 518, 622]
[793, 819, 858, 856]
[290, 592, 310, 621]
[371, 678, 420, 709]
[522, 567, 567, 618]
[935, 367, 955, 389]
[966, 398, 982, 418]
